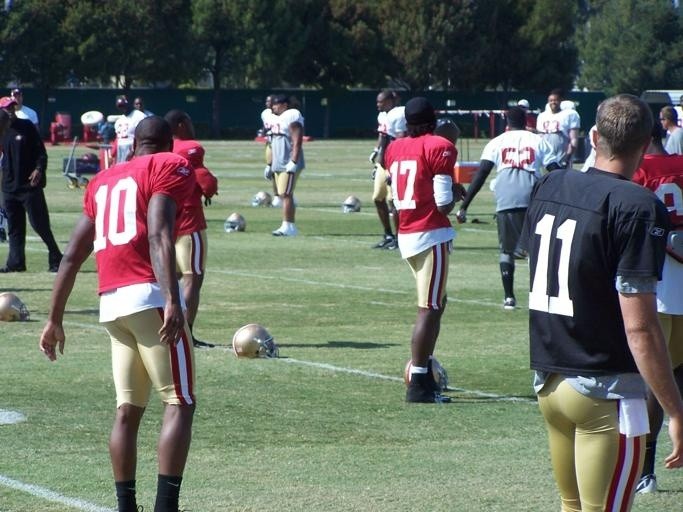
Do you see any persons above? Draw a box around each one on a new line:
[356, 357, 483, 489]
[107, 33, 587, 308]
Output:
[256, 93, 298, 206]
[457, 93, 597, 309]
[372, 91, 410, 251]
[11, 88, 40, 133]
[520, 95, 683, 512]
[370, 90, 402, 215]
[264, 94, 305, 237]
[0, 95, 63, 272]
[383, 96, 465, 405]
[40, 115, 197, 512]
[164, 109, 217, 348]
[109, 95, 155, 167]
[631, 106, 682, 495]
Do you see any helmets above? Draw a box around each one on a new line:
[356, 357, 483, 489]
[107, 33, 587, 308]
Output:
[225, 213, 245, 232]
[234, 324, 279, 358]
[342, 196, 360, 214]
[403, 355, 447, 389]
[0, 292, 29, 321]
[253, 191, 270, 207]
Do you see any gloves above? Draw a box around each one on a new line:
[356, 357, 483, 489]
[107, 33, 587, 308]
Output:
[265, 165, 273, 180]
[286, 160, 297, 173]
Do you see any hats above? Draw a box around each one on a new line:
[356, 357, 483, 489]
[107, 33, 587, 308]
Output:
[405, 97, 435, 124]
[0, 89, 22, 107]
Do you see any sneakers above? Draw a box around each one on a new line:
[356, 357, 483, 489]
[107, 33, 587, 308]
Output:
[273, 222, 297, 236]
[504, 296, 516, 309]
[373, 234, 397, 250]
[407, 360, 450, 403]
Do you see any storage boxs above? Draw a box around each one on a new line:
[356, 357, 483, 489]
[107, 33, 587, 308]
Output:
[455, 161, 480, 184]
